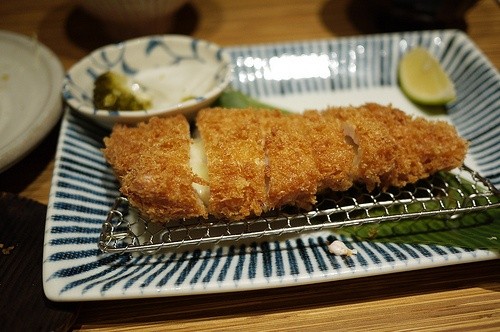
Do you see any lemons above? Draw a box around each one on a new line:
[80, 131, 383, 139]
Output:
[397, 45, 456, 106]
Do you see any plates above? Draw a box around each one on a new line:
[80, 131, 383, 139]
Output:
[62, 35, 232, 125]
[43, 29, 496, 302]
[0, 29, 67, 173]
